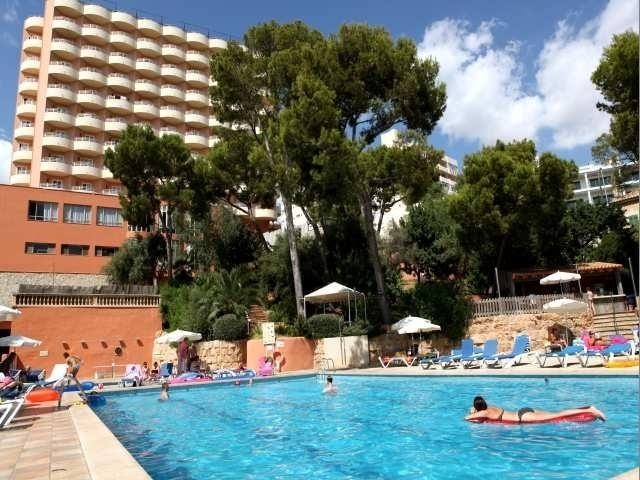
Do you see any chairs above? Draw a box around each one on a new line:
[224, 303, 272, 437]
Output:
[23, 364, 70, 411]
[0, 382, 37, 427]
[378, 328, 640, 370]
[121, 363, 256, 386]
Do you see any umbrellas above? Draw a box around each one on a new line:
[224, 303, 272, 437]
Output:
[397, 321, 442, 343]
[157, 328, 203, 374]
[541, 297, 588, 345]
[1, 335, 42, 370]
[390, 315, 430, 355]
[540, 269, 582, 294]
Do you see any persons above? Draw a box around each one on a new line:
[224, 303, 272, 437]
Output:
[551, 328, 567, 352]
[529, 293, 537, 314]
[586, 287, 597, 316]
[321, 376, 339, 393]
[588, 330, 610, 346]
[421, 337, 437, 357]
[0, 338, 246, 401]
[627, 293, 636, 310]
[465, 395, 607, 422]
